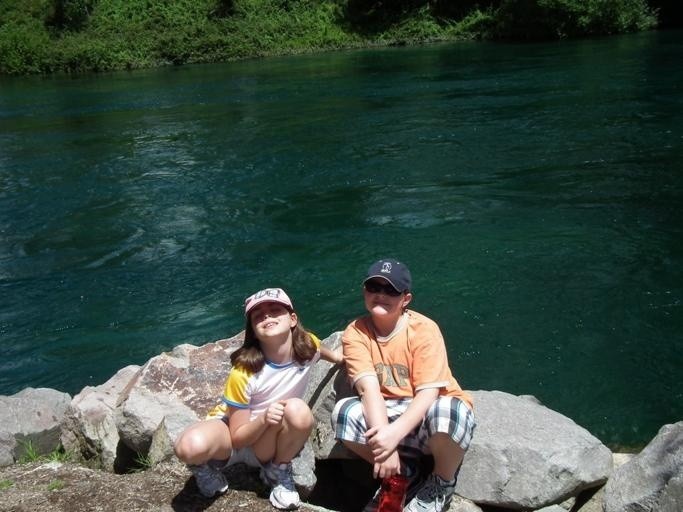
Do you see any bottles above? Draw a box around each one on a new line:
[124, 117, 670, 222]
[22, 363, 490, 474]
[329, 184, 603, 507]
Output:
[374, 473, 412, 512]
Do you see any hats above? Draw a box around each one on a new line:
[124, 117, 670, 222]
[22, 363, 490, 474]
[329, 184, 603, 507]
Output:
[245, 288, 294, 315]
[363, 258, 411, 293]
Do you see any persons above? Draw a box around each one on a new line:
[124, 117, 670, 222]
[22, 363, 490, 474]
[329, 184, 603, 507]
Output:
[331, 258, 477, 512]
[175, 288, 344, 510]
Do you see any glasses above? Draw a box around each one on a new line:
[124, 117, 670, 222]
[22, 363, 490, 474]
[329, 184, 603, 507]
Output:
[365, 279, 402, 296]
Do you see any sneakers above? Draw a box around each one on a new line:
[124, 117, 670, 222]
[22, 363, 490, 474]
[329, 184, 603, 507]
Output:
[371, 468, 423, 507]
[189, 460, 230, 498]
[259, 458, 301, 510]
[403, 473, 457, 512]
